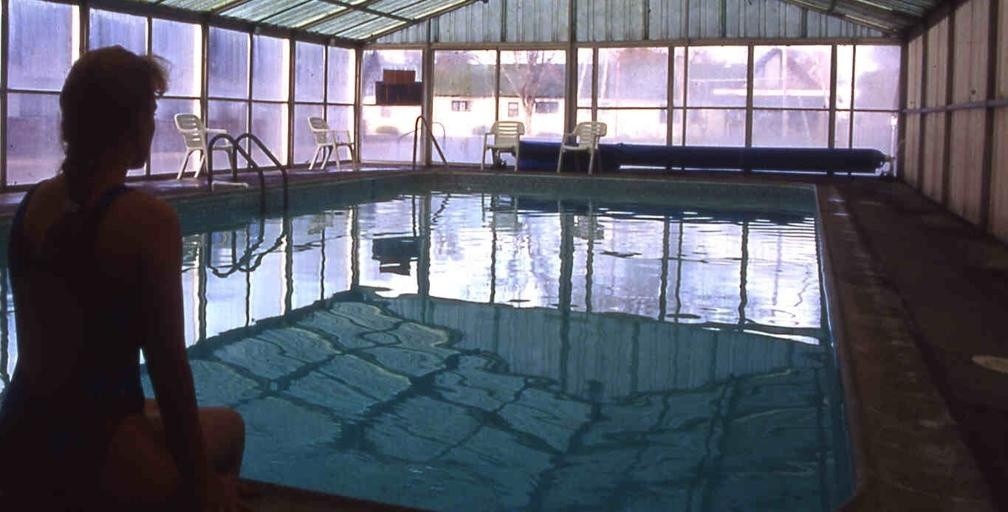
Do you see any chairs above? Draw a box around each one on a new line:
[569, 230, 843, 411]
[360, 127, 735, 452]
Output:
[557, 120, 608, 178]
[482, 120, 526, 174]
[172, 110, 234, 178]
[308, 117, 358, 173]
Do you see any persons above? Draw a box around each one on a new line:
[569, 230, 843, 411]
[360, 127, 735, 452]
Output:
[1, 46, 245, 510]
